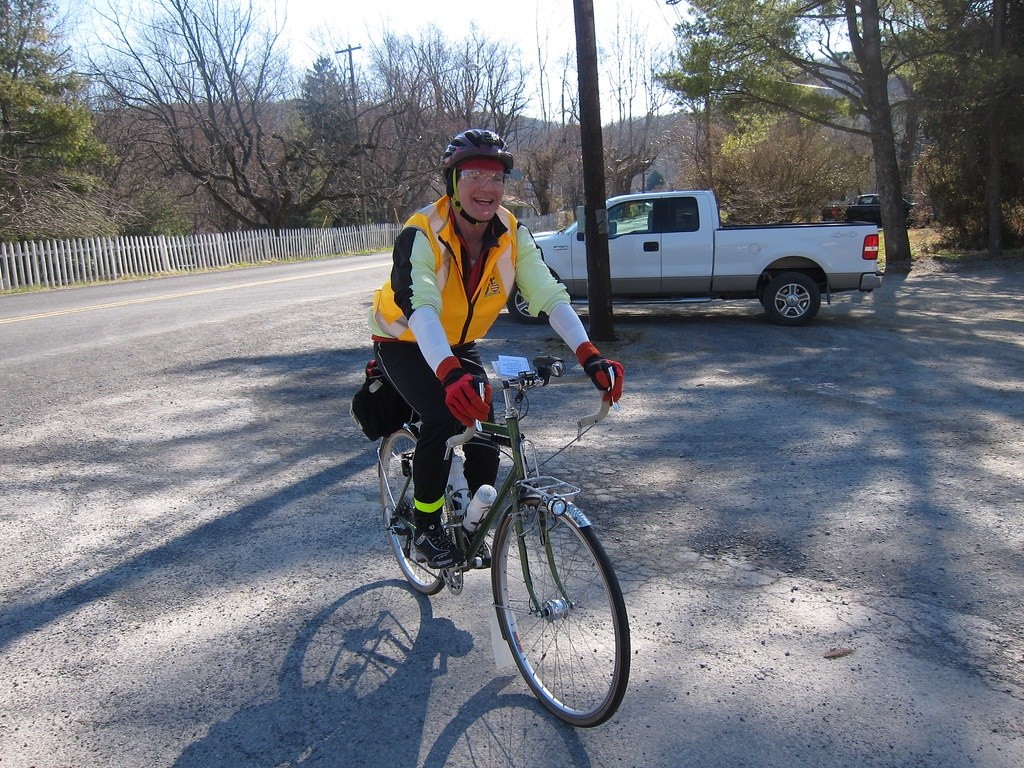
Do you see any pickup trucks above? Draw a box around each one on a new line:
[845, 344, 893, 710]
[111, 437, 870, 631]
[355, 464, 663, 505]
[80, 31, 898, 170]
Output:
[506, 188, 883, 329]
[821, 192, 883, 229]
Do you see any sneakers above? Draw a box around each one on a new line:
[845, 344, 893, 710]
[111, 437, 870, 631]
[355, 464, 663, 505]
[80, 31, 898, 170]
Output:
[413, 528, 459, 569]
[461, 526, 492, 568]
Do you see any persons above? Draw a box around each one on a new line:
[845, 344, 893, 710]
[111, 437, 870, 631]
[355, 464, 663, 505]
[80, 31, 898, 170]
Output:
[365, 130, 625, 570]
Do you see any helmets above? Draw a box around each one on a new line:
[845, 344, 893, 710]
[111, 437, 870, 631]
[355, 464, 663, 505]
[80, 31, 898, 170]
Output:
[439, 129, 514, 174]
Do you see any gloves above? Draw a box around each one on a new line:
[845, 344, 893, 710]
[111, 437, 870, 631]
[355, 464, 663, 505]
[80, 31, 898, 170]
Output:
[576, 341, 623, 405]
[436, 355, 492, 428]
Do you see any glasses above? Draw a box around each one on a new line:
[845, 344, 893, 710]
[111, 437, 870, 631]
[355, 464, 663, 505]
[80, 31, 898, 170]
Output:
[459, 169, 510, 190]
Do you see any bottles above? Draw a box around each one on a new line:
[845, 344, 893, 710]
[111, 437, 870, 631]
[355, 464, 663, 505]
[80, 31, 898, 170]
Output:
[447, 456, 471, 518]
[462, 485, 497, 531]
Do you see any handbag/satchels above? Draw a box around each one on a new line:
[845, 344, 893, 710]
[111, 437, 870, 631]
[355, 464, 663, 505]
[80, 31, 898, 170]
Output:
[349, 360, 421, 441]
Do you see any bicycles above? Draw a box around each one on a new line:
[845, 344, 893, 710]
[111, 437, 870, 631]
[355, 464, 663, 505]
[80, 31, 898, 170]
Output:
[374, 356, 633, 727]
